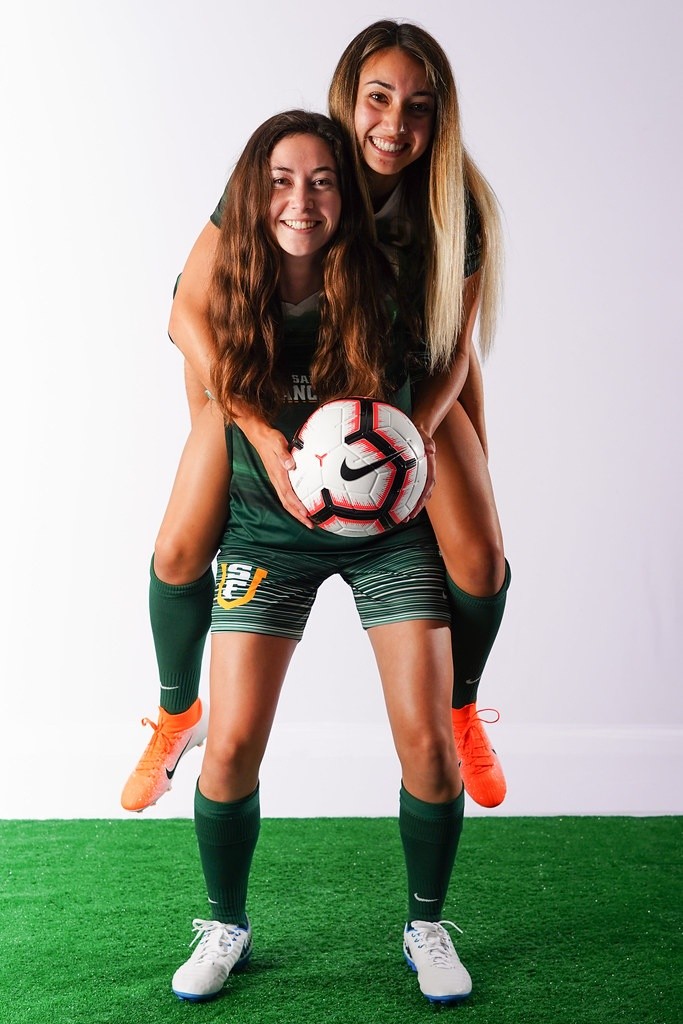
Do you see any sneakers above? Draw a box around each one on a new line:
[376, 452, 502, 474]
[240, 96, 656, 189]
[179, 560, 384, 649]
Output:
[121, 698, 209, 816]
[170, 911, 253, 1001]
[449, 704, 507, 809]
[402, 919, 473, 1002]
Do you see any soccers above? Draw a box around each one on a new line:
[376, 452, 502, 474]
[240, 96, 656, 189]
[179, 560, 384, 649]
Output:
[283, 391, 431, 542]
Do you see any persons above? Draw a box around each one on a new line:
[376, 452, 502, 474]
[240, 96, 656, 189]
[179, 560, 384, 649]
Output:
[119, 14, 510, 809]
[166, 107, 489, 1006]
